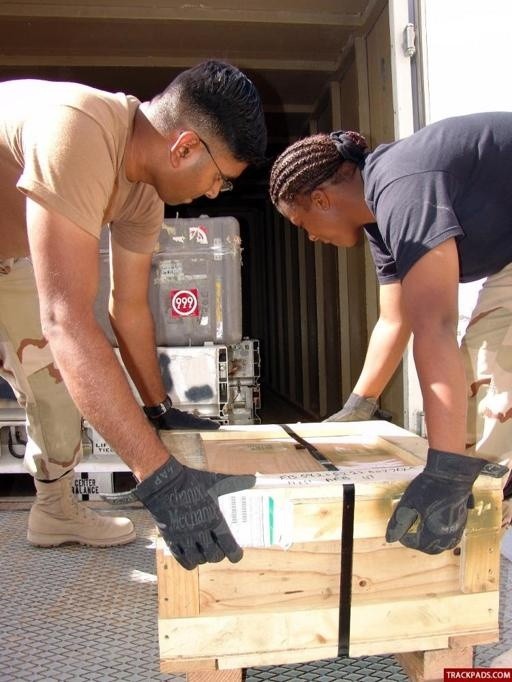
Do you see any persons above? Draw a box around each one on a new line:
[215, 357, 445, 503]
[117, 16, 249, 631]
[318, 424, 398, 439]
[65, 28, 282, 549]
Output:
[1, 56, 270, 571]
[268, 110, 512, 557]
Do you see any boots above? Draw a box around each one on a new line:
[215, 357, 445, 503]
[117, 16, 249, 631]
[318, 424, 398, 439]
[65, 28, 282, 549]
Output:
[24, 467, 137, 549]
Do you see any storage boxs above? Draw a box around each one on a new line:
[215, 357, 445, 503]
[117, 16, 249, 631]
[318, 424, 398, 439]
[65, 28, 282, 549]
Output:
[146, 417, 502, 681]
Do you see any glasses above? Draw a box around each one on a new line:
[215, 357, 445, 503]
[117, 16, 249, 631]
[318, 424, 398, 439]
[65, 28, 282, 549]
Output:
[178, 129, 236, 193]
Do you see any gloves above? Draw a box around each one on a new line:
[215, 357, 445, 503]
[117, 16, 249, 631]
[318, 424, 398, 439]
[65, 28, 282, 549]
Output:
[318, 392, 380, 424]
[146, 407, 221, 432]
[97, 453, 258, 572]
[383, 447, 492, 558]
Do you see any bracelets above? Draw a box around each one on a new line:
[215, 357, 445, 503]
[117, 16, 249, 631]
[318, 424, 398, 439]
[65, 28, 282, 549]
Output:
[141, 395, 170, 420]
